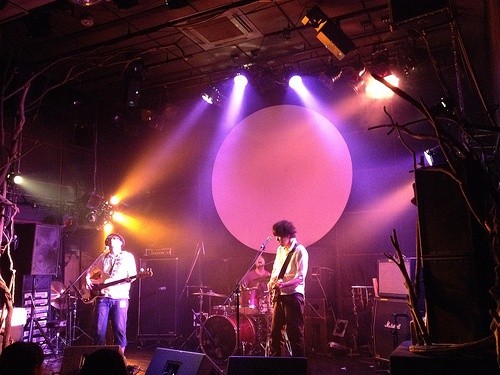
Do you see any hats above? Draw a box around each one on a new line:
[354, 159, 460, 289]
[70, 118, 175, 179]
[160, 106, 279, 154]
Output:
[105, 232, 125, 248]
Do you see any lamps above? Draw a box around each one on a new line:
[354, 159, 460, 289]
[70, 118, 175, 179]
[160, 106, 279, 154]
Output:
[201, 46, 396, 105]
[6, 171, 22, 187]
[424, 142, 450, 166]
[433, 97, 454, 116]
[333, 319, 351, 347]
[62, 193, 127, 234]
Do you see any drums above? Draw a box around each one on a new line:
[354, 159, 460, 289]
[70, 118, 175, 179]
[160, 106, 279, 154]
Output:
[238, 287, 259, 316]
[258, 291, 271, 314]
[200, 313, 255, 362]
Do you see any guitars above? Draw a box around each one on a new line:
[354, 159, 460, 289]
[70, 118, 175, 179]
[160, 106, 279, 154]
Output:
[268, 276, 281, 308]
[81, 267, 153, 304]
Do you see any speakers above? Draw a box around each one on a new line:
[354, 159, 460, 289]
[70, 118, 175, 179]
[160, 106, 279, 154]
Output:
[144, 348, 227, 375]
[226, 355, 311, 375]
[59, 344, 126, 375]
[414, 161, 493, 345]
[18, 222, 61, 276]
[373, 298, 411, 362]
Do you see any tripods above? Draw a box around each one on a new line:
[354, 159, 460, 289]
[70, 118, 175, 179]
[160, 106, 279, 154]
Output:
[24, 275, 57, 359]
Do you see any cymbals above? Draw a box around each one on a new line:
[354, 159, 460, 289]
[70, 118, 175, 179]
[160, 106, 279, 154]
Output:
[246, 275, 271, 288]
[193, 291, 224, 297]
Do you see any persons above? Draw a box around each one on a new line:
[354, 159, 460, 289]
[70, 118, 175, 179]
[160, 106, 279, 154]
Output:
[245, 221, 309, 357]
[80, 349, 131, 375]
[0, 341, 44, 375]
[86, 233, 138, 353]
